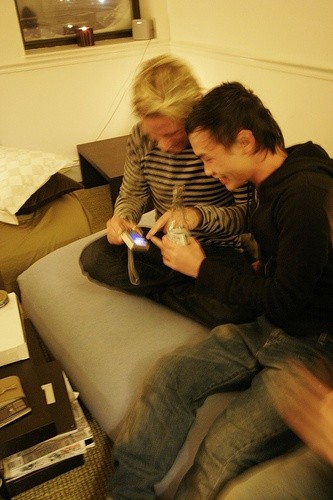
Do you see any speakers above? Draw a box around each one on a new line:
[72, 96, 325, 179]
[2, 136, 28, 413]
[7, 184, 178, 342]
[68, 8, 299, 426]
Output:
[132, 19, 153, 40]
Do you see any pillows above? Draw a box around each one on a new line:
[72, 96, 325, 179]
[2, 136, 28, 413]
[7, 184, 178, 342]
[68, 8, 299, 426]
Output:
[0, 144, 83, 225]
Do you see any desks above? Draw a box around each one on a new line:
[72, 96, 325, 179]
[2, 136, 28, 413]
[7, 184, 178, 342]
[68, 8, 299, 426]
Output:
[77, 134, 129, 211]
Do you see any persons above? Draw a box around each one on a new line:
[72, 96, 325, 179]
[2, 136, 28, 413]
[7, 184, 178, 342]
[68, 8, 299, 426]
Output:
[80, 52, 263, 327]
[107, 82, 333, 500]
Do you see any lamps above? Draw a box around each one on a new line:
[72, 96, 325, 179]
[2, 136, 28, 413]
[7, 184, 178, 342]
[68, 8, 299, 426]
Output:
[76, 26, 94, 46]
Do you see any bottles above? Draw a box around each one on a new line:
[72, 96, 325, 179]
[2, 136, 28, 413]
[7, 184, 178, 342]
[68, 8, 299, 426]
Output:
[166, 183, 191, 245]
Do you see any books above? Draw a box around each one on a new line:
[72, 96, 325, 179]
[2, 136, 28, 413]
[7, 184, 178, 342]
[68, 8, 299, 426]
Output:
[0, 376, 31, 428]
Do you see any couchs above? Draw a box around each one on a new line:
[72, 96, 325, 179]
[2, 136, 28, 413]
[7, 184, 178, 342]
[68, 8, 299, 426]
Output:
[0, 185, 113, 297]
[17, 210, 241, 500]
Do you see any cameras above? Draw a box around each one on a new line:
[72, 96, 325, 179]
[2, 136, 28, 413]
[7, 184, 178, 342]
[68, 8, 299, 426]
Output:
[121, 230, 150, 252]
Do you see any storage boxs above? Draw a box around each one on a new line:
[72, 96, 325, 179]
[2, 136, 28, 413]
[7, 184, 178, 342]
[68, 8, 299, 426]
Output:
[0, 292, 30, 367]
